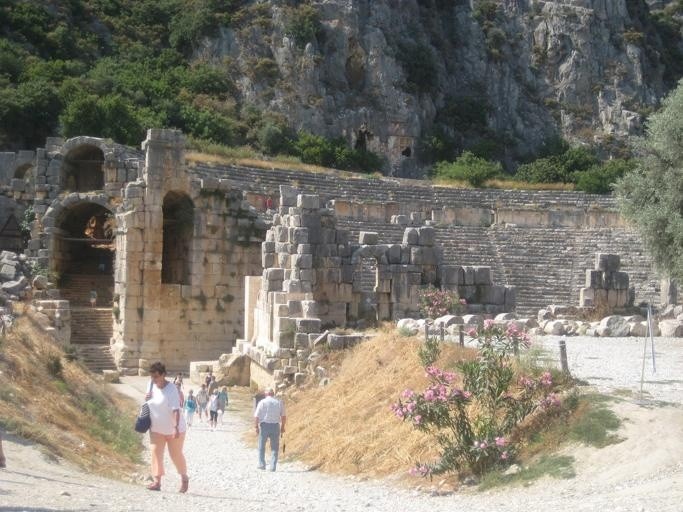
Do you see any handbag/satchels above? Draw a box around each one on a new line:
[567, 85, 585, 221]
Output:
[135, 402, 151, 434]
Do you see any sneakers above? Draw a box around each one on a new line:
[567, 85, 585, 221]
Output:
[179, 475, 189, 493]
[146, 483, 161, 491]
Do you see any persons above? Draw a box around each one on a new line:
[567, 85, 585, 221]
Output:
[89, 287, 97, 308]
[144, 361, 189, 494]
[253, 388, 287, 472]
[173, 371, 229, 432]
